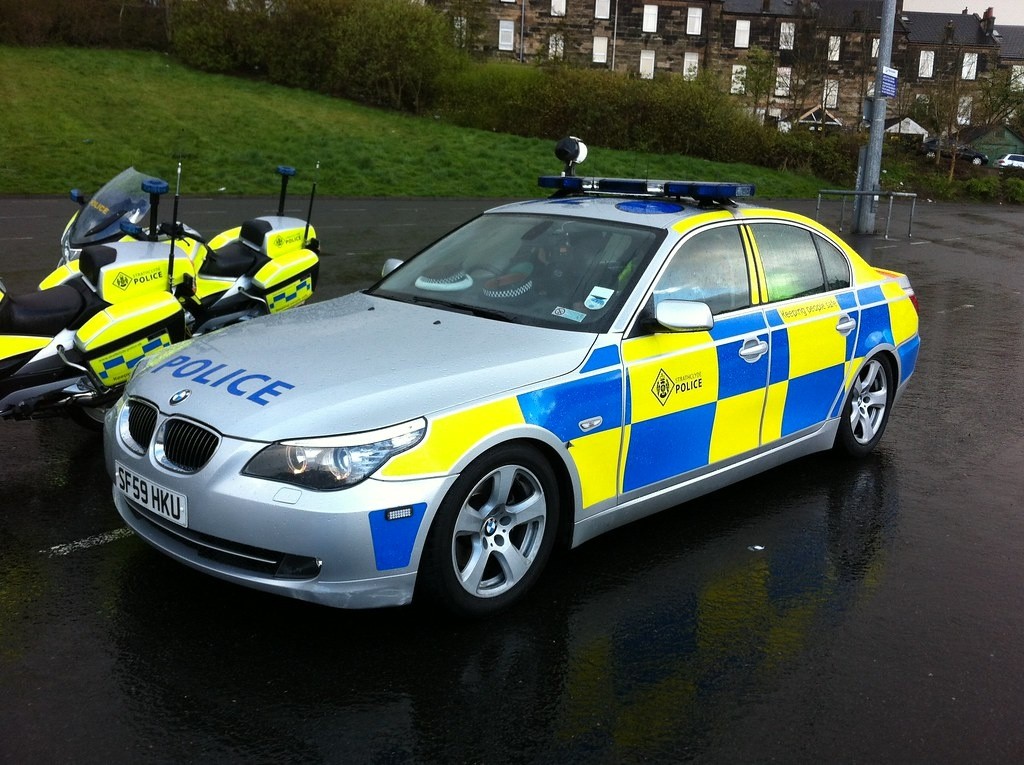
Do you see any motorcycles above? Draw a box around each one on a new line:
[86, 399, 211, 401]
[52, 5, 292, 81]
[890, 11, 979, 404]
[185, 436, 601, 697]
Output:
[55, 165, 322, 337]
[0, 161, 202, 433]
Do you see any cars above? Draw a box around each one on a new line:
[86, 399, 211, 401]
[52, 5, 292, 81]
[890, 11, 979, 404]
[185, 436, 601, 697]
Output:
[922, 138, 988, 166]
[104, 136, 920, 618]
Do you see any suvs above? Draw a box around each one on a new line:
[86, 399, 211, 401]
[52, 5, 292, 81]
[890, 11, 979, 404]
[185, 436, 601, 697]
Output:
[993, 154, 1024, 171]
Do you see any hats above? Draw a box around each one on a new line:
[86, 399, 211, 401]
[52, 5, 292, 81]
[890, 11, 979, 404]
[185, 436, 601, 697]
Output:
[413, 265, 473, 293]
[479, 273, 535, 306]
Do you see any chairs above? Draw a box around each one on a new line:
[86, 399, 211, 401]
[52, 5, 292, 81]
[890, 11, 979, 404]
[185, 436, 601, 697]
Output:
[545, 222, 613, 300]
